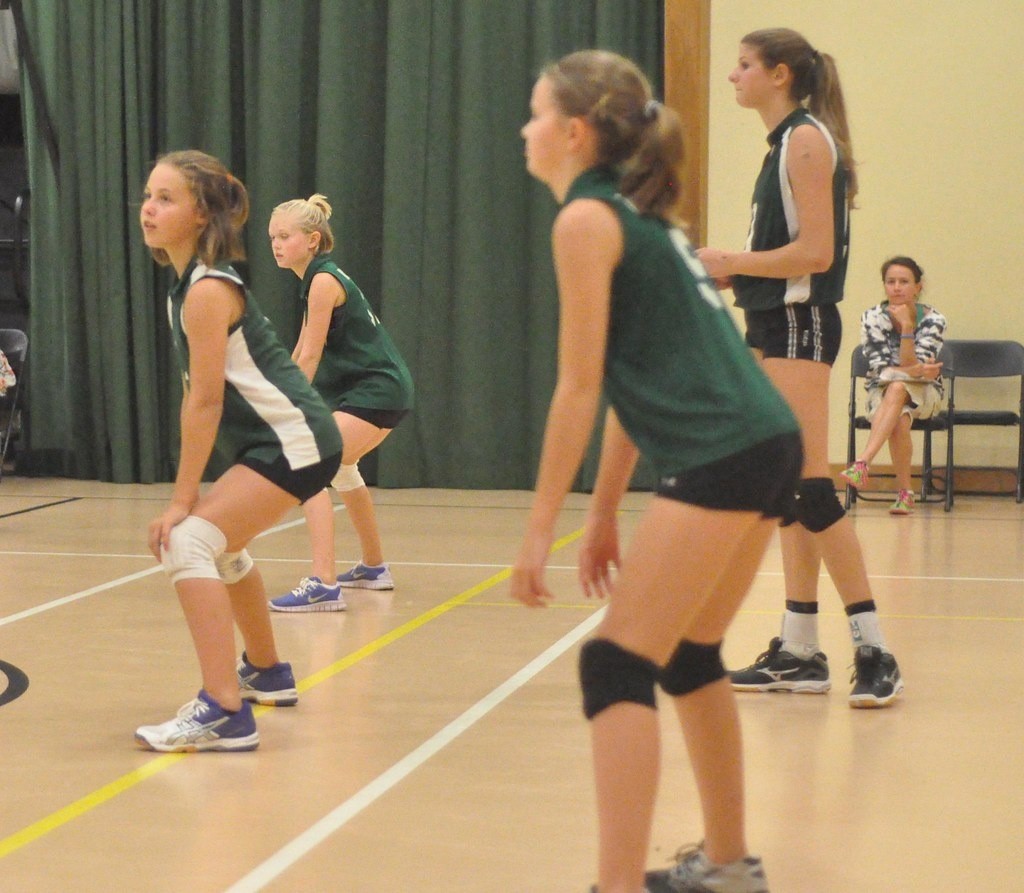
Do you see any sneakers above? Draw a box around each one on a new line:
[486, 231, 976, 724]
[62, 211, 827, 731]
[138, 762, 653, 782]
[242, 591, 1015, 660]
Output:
[646, 842, 769, 893]
[135, 690, 259, 752]
[841, 460, 869, 488]
[891, 489, 914, 514]
[848, 645, 904, 707]
[337, 562, 393, 590]
[726, 637, 830, 693]
[237, 658, 298, 705]
[268, 576, 347, 611]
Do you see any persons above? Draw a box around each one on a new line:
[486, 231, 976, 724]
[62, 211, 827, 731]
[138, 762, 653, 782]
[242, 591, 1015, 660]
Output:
[267, 192, 418, 612]
[506, 48, 806, 893]
[127, 150, 344, 754]
[838, 253, 946, 514]
[690, 26, 905, 710]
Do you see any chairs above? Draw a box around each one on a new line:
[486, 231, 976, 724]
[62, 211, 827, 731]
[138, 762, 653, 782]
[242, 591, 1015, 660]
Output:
[0, 329, 28, 476]
[920, 339, 1024, 507]
[845, 340, 955, 513]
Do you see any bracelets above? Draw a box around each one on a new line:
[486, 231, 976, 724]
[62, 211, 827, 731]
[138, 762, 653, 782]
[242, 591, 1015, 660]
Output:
[900, 334, 915, 339]
